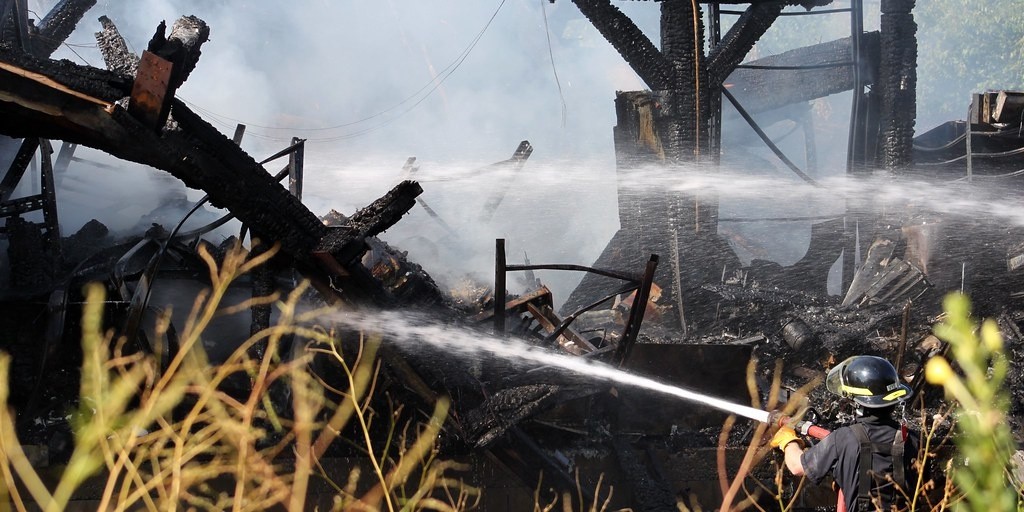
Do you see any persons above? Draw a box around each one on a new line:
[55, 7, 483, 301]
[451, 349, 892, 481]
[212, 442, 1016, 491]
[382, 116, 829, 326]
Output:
[770, 355, 931, 512]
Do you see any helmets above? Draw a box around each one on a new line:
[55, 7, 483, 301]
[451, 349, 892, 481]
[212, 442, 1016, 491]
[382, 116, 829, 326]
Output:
[843, 357, 913, 408]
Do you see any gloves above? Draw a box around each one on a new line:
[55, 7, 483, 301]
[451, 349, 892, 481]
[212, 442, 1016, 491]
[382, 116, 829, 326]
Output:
[770, 424, 806, 450]
